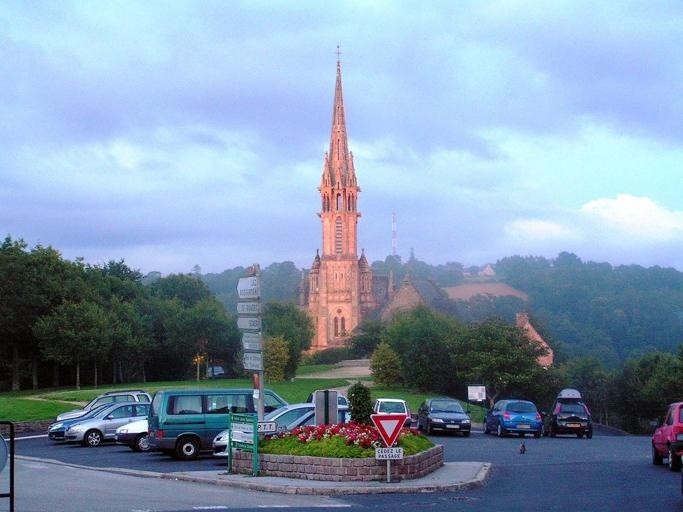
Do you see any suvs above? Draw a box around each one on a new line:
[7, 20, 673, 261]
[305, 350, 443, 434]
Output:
[56, 389, 153, 422]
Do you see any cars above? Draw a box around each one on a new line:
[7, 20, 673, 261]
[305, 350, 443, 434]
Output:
[64, 402, 151, 448]
[47, 401, 122, 442]
[652, 402, 683, 472]
[211, 403, 352, 460]
[208, 366, 234, 379]
[370, 398, 412, 427]
[306, 389, 349, 406]
[115, 418, 150, 452]
[418, 398, 472, 437]
[484, 388, 593, 438]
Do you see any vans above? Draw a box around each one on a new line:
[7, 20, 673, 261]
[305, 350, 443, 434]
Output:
[146, 387, 291, 462]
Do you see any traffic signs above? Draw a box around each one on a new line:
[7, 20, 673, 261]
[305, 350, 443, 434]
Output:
[237, 263, 264, 371]
